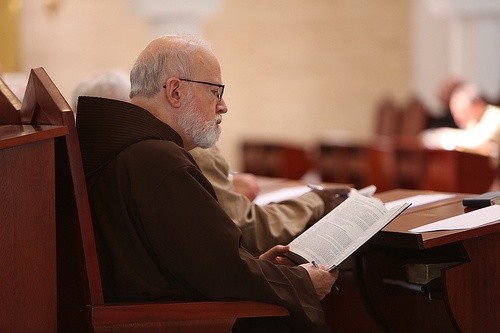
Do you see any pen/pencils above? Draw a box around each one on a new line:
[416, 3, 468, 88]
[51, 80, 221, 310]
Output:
[312, 261, 339, 291]
[308, 184, 346, 198]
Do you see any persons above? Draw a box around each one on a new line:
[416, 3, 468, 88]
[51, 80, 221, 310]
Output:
[72, 71, 351, 256]
[76, 35, 339, 333]
[418, 78, 500, 158]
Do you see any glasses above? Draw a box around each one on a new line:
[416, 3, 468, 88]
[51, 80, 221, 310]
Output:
[163, 78, 225, 102]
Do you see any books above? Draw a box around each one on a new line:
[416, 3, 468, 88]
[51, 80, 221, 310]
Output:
[285, 193, 413, 272]
[462, 191, 500, 208]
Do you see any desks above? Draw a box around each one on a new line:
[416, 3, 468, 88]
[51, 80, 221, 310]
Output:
[241, 142, 500, 333]
[0, 125, 68, 333]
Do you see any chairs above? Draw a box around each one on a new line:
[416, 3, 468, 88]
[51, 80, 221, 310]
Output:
[0, 67, 289, 333]
[375, 100, 426, 146]
